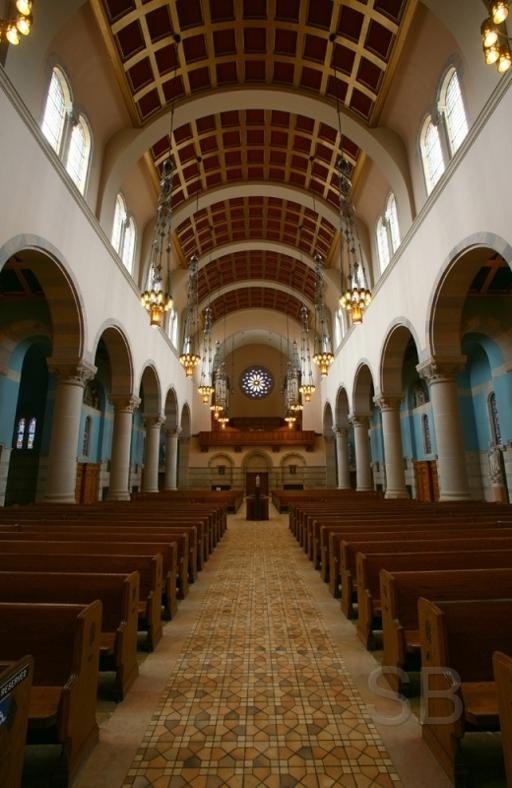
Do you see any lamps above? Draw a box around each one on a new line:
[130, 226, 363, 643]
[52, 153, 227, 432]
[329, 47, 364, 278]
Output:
[0, 0, 34, 45]
[140, 32, 230, 429]
[481, 0, 512, 74]
[284, 34, 371, 429]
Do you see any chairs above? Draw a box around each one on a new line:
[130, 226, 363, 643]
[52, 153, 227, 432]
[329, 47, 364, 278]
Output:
[271, 489, 512, 788]
[0, 489, 244, 788]
